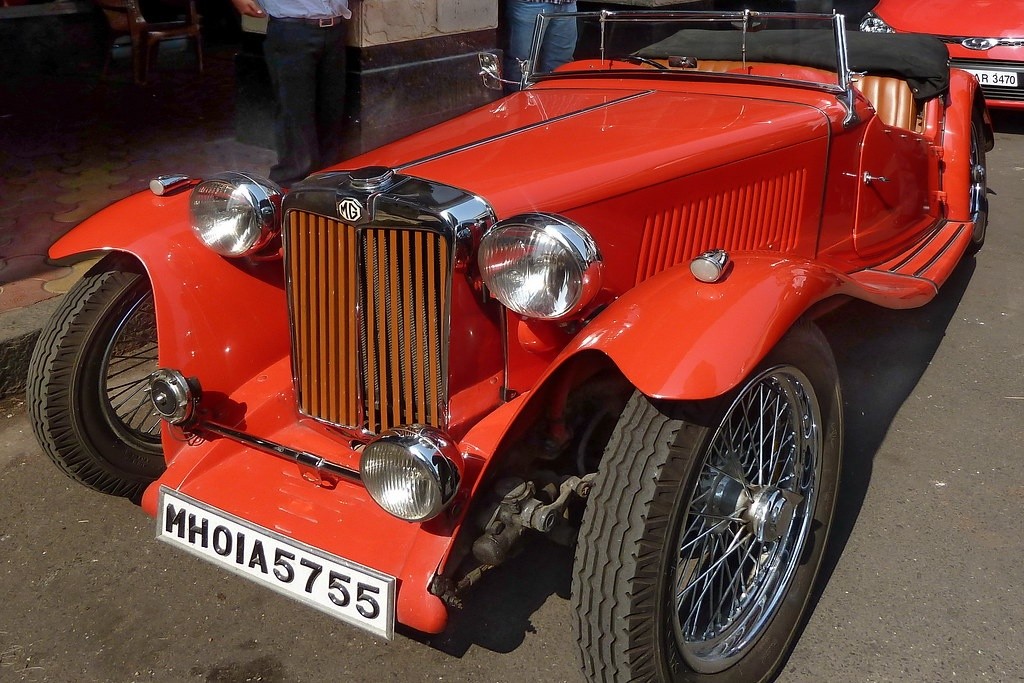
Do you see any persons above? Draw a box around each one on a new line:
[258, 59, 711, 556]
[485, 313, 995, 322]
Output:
[228, 0, 352, 180]
[503, 0, 577, 93]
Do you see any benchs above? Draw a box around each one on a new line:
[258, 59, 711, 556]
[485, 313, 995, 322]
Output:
[639, 58, 917, 132]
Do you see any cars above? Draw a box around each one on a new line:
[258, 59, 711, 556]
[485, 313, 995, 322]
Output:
[28, 10, 994, 683]
[860, 0, 1024, 113]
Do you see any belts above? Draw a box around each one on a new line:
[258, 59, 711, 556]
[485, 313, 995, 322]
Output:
[275, 17, 343, 28]
